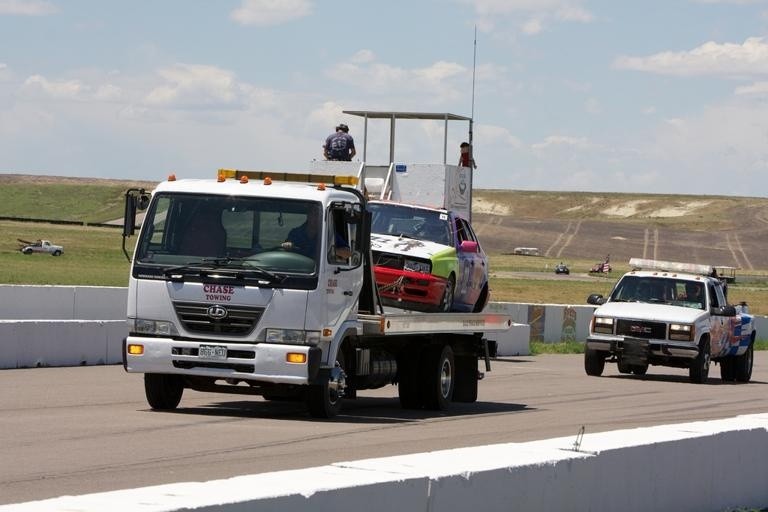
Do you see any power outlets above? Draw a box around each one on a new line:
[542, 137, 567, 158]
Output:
[581, 257, 756, 384]
[20, 239, 65, 257]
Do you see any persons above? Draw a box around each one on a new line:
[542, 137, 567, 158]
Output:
[281, 205, 350, 263]
[678, 282, 703, 309]
[628, 282, 657, 301]
[322, 123, 355, 161]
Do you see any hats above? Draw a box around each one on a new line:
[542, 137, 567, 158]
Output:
[358, 196, 490, 315]
[554, 264, 569, 275]
[588, 263, 612, 273]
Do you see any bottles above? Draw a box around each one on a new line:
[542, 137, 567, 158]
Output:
[336, 124, 349, 132]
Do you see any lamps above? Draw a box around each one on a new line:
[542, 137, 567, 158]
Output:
[178, 203, 226, 257]
